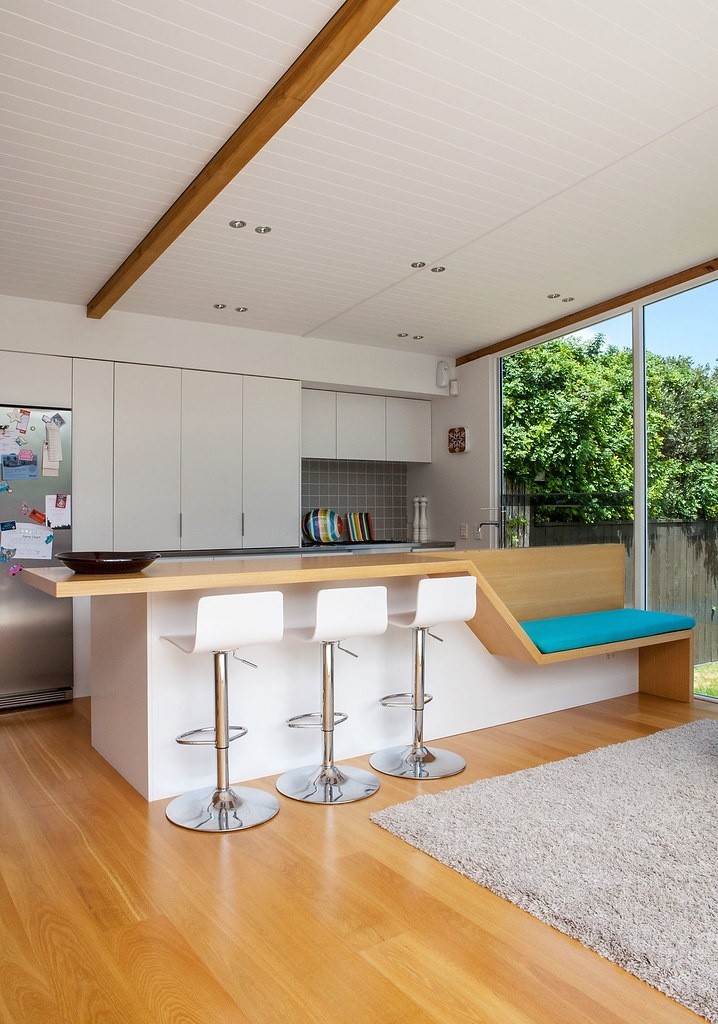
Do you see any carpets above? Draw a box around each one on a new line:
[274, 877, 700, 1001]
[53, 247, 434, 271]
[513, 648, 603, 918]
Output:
[369, 718, 718, 1024]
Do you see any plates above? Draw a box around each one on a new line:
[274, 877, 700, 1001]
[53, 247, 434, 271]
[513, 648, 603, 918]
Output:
[53, 553, 160, 575]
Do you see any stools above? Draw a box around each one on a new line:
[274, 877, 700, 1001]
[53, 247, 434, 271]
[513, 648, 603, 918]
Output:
[158, 590, 288, 835]
[275, 586, 389, 805]
[370, 575, 478, 776]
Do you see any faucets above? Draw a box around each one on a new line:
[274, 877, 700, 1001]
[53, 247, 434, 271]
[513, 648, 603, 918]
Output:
[478, 506, 506, 549]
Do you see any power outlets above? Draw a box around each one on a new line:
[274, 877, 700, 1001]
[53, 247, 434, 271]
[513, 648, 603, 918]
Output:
[459, 523, 468, 539]
[473, 523, 483, 542]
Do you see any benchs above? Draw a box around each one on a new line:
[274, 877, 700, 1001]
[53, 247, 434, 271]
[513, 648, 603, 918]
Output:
[511, 597, 696, 705]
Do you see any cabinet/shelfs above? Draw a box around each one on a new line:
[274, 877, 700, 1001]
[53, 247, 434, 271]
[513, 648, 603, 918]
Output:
[0, 348, 433, 554]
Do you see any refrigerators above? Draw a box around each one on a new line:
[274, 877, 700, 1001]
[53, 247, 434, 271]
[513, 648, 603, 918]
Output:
[1, 405, 71, 712]
